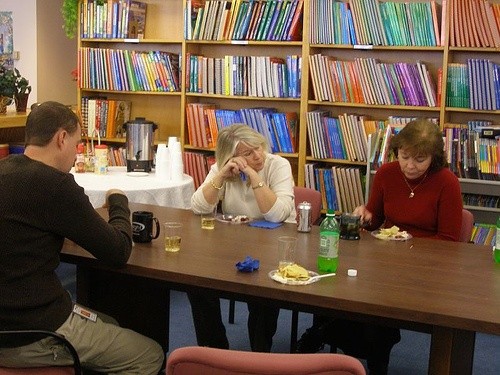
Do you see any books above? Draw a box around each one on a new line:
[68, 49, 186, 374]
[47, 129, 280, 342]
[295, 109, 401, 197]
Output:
[446, 58, 500, 110]
[443, 119, 500, 181]
[471, 223, 497, 246]
[185, 102, 298, 153]
[449, 0, 500, 47]
[307, 109, 439, 169]
[462, 193, 500, 208]
[78, 0, 183, 167]
[184, 0, 304, 41]
[305, 163, 366, 214]
[308, 54, 444, 107]
[310, 0, 440, 46]
[183, 150, 216, 190]
[186, 52, 302, 97]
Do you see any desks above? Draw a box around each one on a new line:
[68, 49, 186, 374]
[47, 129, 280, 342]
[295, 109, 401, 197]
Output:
[0, 108, 31, 143]
[60, 203, 500, 375]
[69, 166, 195, 209]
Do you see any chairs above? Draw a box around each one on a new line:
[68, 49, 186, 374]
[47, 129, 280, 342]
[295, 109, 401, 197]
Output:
[0, 330, 81, 375]
[330, 209, 473, 352]
[229, 186, 323, 352]
[165, 346, 366, 375]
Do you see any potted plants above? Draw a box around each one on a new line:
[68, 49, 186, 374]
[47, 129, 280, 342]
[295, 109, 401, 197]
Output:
[13, 68, 31, 112]
[0, 64, 12, 112]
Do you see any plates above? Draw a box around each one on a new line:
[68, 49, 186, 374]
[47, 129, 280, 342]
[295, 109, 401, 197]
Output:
[269, 270, 321, 286]
[373, 230, 413, 241]
[217, 214, 253, 225]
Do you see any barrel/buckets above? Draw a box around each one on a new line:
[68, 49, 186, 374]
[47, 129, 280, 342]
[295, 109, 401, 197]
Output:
[120, 117, 157, 174]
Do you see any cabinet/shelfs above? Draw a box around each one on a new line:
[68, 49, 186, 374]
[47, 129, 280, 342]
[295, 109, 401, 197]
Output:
[77, 0, 500, 216]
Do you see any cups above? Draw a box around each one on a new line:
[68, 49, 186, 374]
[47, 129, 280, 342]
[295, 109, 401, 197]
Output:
[277, 236, 298, 271]
[200, 204, 217, 231]
[131, 211, 161, 244]
[155, 137, 183, 181]
[163, 223, 182, 252]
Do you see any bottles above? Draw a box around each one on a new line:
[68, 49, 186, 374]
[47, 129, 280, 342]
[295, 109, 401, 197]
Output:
[316, 210, 340, 274]
[94, 145, 108, 174]
[319, 209, 361, 240]
[493, 216, 500, 263]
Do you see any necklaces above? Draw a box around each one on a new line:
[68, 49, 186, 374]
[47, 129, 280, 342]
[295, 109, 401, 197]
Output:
[402, 174, 426, 197]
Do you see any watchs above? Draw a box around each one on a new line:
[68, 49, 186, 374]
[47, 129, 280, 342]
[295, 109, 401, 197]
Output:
[252, 182, 264, 189]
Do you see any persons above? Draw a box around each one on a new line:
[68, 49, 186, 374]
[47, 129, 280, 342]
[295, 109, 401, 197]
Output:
[81, 310, 90, 317]
[187, 124, 298, 352]
[293, 119, 463, 375]
[0, 101, 164, 375]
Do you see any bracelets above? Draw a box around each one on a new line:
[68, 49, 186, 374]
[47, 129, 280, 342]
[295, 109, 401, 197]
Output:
[210, 178, 223, 189]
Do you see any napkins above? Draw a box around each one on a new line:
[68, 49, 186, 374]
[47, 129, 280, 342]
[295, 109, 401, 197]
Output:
[234, 256, 260, 273]
[248, 220, 285, 230]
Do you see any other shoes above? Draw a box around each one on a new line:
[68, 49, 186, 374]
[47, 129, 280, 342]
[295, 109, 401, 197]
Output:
[295, 327, 326, 354]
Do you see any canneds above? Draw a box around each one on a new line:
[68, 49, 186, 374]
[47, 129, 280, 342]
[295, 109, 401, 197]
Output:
[95, 144, 108, 175]
[295, 200, 312, 233]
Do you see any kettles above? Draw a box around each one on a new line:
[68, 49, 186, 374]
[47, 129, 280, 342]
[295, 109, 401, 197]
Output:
[15, 92, 28, 114]
